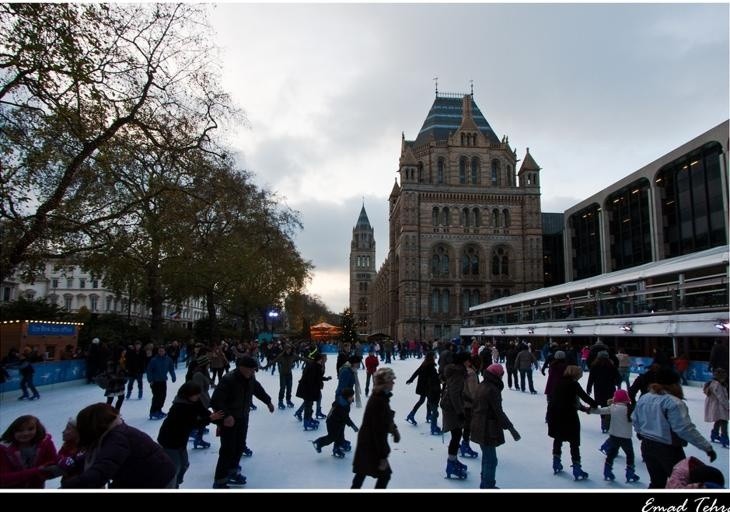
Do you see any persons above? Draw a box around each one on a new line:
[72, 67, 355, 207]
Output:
[546, 351, 568, 423]
[645, 346, 667, 371]
[674, 457, 724, 489]
[441, 352, 470, 477]
[56, 419, 88, 488]
[471, 363, 511, 488]
[629, 369, 716, 489]
[350, 371, 400, 488]
[211, 356, 274, 489]
[1, 415, 59, 489]
[549, 365, 598, 476]
[597, 391, 639, 479]
[61, 402, 176, 489]
[158, 381, 225, 489]
[461, 285, 628, 327]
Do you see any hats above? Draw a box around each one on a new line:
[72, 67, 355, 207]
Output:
[372, 366, 398, 386]
[236, 353, 260, 370]
[551, 349, 568, 360]
[612, 387, 631, 404]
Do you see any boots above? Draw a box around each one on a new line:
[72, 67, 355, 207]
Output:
[623, 471, 642, 480]
[570, 466, 591, 478]
[602, 469, 618, 480]
[552, 457, 564, 471]
[294, 407, 327, 429]
[227, 472, 248, 485]
[333, 439, 351, 457]
[194, 437, 210, 447]
[148, 409, 166, 419]
[313, 439, 326, 455]
[405, 415, 418, 425]
[278, 399, 294, 410]
[445, 458, 471, 478]
[425, 414, 444, 433]
[709, 431, 729, 447]
